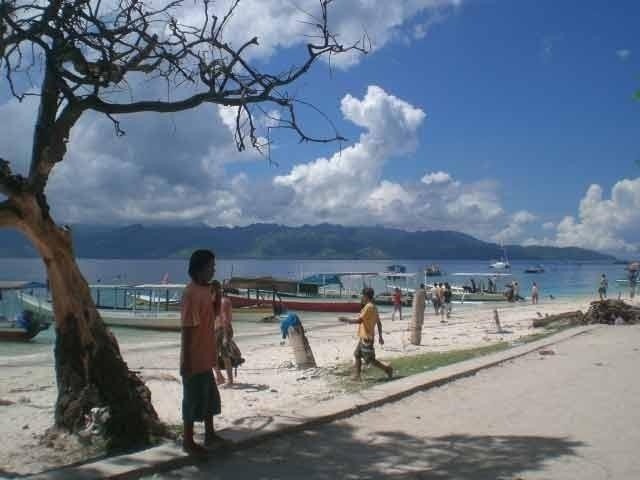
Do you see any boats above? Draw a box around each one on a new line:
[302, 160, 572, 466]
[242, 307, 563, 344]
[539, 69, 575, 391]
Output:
[130, 281, 186, 306]
[0, 280, 50, 342]
[616, 276, 640, 286]
[223, 264, 512, 315]
[17, 283, 181, 332]
[491, 260, 510, 269]
[526, 265, 544, 274]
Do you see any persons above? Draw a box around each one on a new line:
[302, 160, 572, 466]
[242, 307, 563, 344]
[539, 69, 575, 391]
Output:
[210, 278, 236, 388]
[177, 249, 233, 454]
[391, 287, 403, 321]
[338, 287, 394, 380]
[418, 278, 540, 324]
[598, 270, 610, 301]
